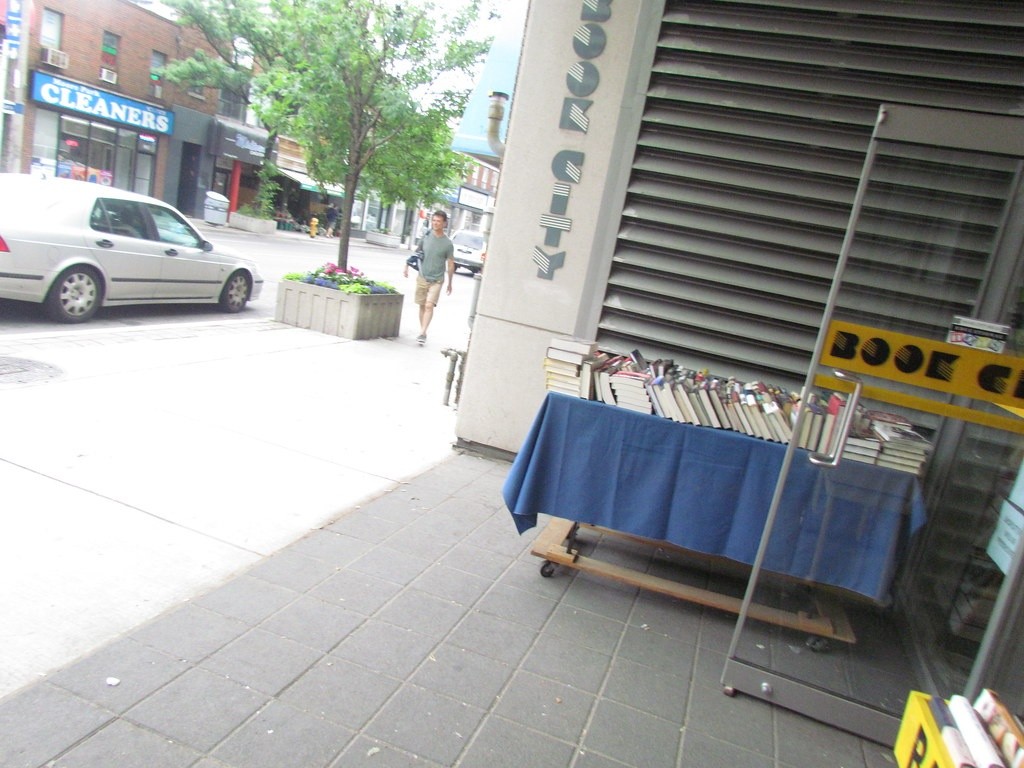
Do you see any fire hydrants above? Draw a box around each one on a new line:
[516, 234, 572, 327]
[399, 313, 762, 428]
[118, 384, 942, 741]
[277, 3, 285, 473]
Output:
[309, 218, 319, 238]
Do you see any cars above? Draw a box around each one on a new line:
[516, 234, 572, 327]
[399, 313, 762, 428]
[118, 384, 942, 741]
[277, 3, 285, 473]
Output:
[1, 174, 264, 325]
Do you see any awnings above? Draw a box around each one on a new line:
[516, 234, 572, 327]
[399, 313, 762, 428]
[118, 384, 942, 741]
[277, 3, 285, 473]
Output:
[277, 168, 346, 198]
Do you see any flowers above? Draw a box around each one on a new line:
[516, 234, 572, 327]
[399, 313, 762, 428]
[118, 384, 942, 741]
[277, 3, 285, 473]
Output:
[284, 262, 397, 296]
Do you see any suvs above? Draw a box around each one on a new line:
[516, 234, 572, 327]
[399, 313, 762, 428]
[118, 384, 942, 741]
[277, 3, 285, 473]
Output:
[446, 227, 487, 273]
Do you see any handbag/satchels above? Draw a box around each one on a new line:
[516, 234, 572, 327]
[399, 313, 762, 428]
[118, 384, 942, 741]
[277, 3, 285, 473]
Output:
[406, 230, 430, 271]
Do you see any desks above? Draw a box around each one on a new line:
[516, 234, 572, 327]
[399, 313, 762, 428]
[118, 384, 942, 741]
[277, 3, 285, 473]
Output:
[501, 392, 926, 650]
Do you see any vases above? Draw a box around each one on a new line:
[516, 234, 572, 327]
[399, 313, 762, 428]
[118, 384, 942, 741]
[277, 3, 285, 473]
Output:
[277, 281, 403, 341]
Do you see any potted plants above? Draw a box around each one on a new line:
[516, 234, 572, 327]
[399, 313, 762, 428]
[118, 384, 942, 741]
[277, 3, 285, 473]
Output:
[229, 160, 279, 233]
[367, 228, 401, 247]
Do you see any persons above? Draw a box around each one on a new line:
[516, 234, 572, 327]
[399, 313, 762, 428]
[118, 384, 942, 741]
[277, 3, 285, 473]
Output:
[403, 210, 454, 343]
[325, 204, 339, 239]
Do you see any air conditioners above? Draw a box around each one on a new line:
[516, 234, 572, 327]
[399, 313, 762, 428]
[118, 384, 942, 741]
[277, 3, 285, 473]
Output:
[99, 69, 118, 85]
[40, 48, 68, 70]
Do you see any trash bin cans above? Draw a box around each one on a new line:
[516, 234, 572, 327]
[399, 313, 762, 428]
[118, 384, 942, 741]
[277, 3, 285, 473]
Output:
[204, 190, 230, 226]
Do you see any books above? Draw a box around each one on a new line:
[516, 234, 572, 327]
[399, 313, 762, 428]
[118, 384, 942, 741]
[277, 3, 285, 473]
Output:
[929, 689, 1024, 768]
[542, 335, 935, 475]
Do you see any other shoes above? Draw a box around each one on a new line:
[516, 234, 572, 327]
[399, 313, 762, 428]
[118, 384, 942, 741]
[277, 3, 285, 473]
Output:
[416, 335, 426, 344]
